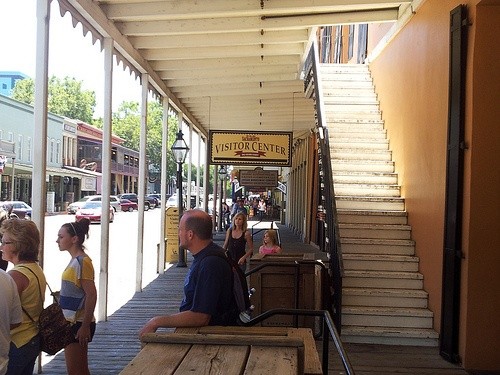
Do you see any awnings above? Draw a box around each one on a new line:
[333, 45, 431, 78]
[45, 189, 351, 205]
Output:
[4, 163, 83, 182]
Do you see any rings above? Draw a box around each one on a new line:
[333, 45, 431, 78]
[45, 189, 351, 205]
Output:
[86, 338, 89, 339]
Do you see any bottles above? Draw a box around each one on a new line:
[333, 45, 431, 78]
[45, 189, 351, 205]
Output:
[50, 291, 61, 306]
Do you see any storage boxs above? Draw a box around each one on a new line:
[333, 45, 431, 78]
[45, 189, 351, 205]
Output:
[120, 326, 324, 375]
[249, 253, 316, 338]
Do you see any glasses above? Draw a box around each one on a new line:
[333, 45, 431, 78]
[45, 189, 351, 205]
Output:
[1, 240, 17, 245]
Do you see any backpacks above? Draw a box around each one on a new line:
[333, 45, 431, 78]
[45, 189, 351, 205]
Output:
[37, 304, 74, 356]
[196, 250, 250, 323]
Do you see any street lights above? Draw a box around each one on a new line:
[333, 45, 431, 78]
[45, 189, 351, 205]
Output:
[169, 130, 190, 267]
[216, 165, 227, 234]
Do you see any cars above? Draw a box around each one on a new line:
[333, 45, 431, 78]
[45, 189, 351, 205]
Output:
[119, 199, 138, 212]
[67, 195, 120, 214]
[75, 201, 115, 223]
[0, 201, 32, 222]
[117, 193, 217, 211]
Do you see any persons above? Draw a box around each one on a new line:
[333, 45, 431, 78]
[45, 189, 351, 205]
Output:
[0, 207, 46, 375]
[259, 230, 280, 254]
[57, 218, 98, 375]
[137, 209, 246, 343]
[222, 194, 272, 230]
[222, 211, 253, 272]
